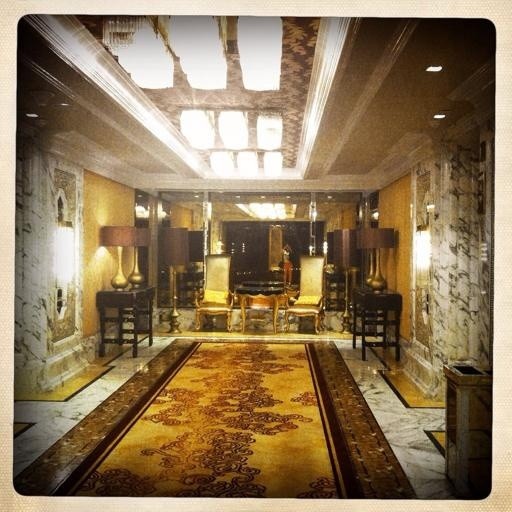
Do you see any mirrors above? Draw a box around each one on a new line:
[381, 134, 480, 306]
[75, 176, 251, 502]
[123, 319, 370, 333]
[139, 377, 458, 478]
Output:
[157, 192, 361, 307]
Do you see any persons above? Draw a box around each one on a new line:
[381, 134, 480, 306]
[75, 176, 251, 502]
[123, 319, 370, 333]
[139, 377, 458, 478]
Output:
[280, 244, 293, 287]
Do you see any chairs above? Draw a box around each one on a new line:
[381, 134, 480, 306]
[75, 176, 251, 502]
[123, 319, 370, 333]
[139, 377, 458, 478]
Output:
[194, 254, 235, 331]
[284, 254, 327, 333]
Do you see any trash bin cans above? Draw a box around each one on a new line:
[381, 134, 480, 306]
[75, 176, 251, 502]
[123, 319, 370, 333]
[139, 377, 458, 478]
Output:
[443, 364, 492, 500]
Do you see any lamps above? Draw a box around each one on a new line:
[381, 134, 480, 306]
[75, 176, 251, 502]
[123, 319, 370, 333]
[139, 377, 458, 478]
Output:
[159, 226, 191, 333]
[332, 226, 359, 332]
[356, 227, 395, 291]
[98, 224, 149, 289]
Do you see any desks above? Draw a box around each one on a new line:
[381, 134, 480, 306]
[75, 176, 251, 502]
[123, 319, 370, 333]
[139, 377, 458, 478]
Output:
[351, 288, 401, 361]
[237, 283, 282, 333]
[93, 290, 156, 357]
